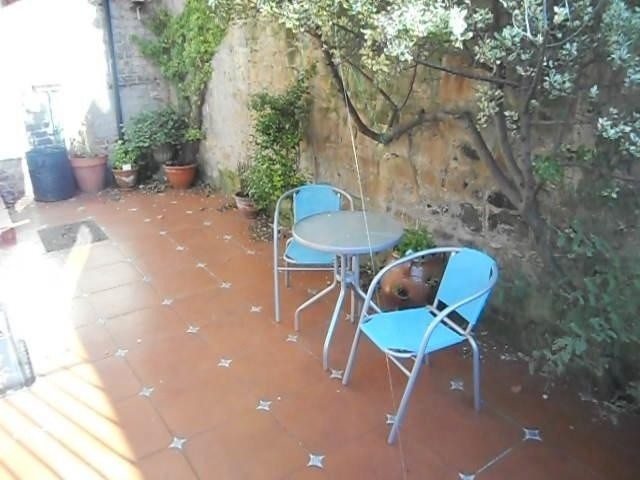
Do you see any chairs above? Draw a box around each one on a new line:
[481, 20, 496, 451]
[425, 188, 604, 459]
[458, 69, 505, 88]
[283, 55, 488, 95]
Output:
[274, 184, 356, 323]
[341, 245, 500, 444]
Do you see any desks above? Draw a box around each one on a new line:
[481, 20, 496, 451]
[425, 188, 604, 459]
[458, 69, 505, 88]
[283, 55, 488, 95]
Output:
[291, 210, 404, 371]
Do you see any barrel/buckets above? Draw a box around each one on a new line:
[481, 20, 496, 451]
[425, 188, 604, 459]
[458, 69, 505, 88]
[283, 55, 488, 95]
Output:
[25, 143, 73, 203]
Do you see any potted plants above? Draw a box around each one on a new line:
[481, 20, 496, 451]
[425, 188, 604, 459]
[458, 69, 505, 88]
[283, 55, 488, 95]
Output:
[378, 222, 447, 314]
[232, 156, 261, 218]
[67, 105, 206, 195]
